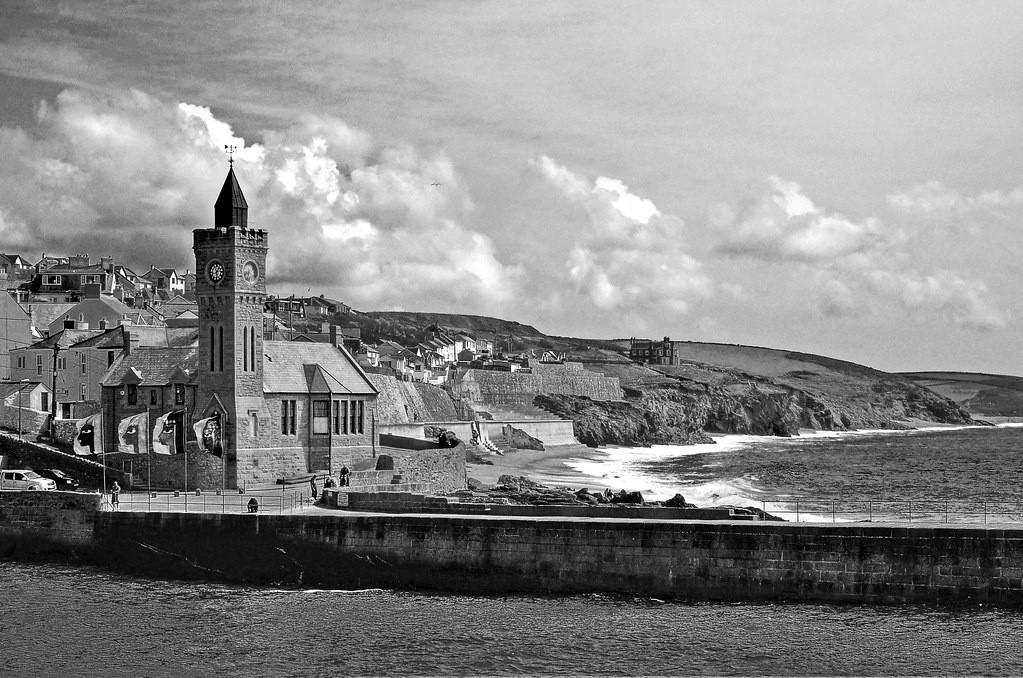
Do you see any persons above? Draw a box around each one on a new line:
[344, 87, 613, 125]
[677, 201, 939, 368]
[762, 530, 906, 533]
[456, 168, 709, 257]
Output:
[603, 488, 614, 498]
[339, 465, 350, 486]
[111, 482, 121, 503]
[247, 497, 258, 512]
[438, 431, 446, 448]
[311, 474, 318, 500]
[325, 477, 337, 487]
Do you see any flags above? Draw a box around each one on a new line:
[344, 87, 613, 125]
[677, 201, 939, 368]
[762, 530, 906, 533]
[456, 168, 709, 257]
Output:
[118, 413, 147, 455]
[153, 410, 185, 455]
[193, 416, 223, 459]
[74, 413, 102, 455]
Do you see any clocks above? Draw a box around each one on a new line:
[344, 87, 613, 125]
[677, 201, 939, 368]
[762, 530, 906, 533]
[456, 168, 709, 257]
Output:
[242, 263, 256, 283]
[208, 264, 224, 283]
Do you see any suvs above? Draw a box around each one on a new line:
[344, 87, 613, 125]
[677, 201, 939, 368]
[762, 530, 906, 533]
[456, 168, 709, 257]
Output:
[31, 468, 80, 491]
[0, 470, 57, 491]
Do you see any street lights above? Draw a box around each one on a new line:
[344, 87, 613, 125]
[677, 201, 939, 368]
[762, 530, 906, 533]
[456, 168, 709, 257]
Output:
[19, 378, 30, 439]
